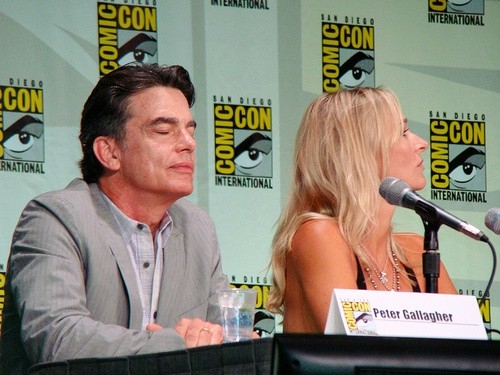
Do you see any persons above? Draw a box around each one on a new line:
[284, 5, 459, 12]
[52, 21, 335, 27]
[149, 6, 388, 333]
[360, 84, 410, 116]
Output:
[265, 83, 459, 332]
[1, 62, 261, 375]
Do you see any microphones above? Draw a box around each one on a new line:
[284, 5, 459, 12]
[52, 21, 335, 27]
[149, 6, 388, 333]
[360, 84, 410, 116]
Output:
[379, 177, 489, 242]
[485, 207, 500, 236]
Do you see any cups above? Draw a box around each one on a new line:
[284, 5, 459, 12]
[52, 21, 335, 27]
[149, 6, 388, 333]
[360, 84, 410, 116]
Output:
[215, 289, 256, 344]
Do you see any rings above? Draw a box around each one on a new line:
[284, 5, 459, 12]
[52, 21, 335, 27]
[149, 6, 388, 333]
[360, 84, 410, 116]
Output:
[200, 328, 212, 334]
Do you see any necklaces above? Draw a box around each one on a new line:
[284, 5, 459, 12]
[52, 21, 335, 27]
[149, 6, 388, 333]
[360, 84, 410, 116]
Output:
[360, 241, 391, 284]
[361, 251, 400, 295]
